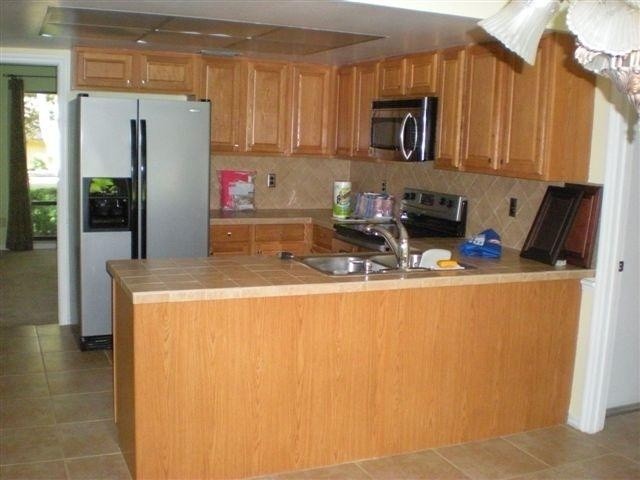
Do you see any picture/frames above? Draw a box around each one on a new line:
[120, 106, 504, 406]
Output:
[519, 186, 586, 265]
[557, 181, 605, 268]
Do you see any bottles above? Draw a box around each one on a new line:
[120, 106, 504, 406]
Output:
[353, 191, 394, 220]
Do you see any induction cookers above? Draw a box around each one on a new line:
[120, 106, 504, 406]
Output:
[332, 187, 469, 241]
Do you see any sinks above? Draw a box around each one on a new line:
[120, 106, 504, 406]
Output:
[292, 255, 395, 278]
[369, 250, 479, 271]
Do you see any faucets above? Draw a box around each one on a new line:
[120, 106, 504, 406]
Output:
[370, 218, 409, 271]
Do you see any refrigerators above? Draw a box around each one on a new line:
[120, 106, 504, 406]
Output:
[69, 97, 209, 352]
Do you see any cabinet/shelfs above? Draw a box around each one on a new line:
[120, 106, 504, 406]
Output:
[465, 31, 598, 184]
[71, 44, 202, 94]
[291, 64, 334, 158]
[312, 221, 377, 253]
[208, 216, 312, 255]
[202, 57, 290, 157]
[334, 59, 380, 159]
[380, 49, 438, 97]
[436, 41, 464, 171]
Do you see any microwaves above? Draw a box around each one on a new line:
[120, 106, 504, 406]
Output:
[367, 96, 436, 163]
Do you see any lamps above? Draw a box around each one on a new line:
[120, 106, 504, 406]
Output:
[476, 1, 640, 118]
